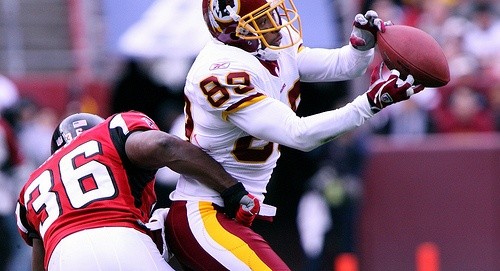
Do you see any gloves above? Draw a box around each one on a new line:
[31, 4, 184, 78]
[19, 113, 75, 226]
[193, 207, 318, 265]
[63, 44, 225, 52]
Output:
[350, 9, 394, 51]
[220, 182, 260, 227]
[366, 60, 424, 114]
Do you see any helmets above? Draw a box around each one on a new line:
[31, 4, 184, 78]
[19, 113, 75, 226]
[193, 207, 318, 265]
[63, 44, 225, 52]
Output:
[202, 0, 277, 53]
[51, 113, 106, 156]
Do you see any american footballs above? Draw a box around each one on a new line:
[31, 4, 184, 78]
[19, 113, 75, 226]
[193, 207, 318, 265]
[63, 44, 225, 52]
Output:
[377, 25, 451, 88]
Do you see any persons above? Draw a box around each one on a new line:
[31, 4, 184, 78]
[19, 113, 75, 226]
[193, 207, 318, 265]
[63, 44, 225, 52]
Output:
[12, 111, 260, 271]
[0, 73, 62, 271]
[159, 0, 425, 271]
[109, 0, 500, 141]
